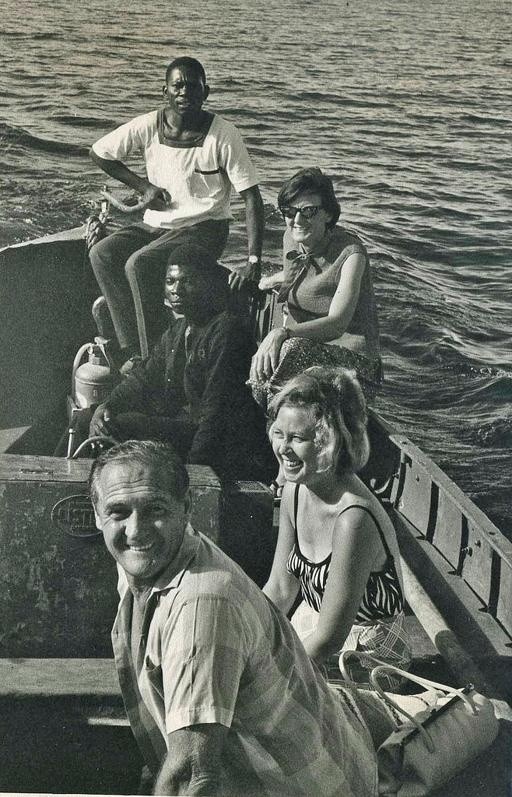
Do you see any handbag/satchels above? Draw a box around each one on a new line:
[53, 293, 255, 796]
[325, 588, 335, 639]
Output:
[336, 648, 503, 797]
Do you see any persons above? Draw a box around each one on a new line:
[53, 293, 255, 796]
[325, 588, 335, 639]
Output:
[50, 241, 263, 472]
[87, 438, 385, 795]
[252, 364, 437, 692]
[244, 160, 384, 408]
[88, 57, 268, 362]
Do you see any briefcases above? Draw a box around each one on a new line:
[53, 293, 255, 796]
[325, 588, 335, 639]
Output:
[0, 435, 222, 660]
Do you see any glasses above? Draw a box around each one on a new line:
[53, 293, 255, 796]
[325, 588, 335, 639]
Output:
[278, 202, 327, 219]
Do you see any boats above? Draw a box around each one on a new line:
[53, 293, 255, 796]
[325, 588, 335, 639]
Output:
[1, 215, 510, 797]
